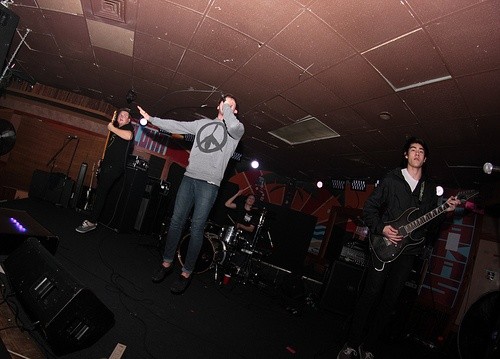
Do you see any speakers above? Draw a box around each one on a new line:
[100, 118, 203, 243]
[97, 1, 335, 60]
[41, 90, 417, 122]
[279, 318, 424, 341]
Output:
[31, 169, 74, 209]
[0, 236, 116, 359]
[0, 207, 60, 263]
[96, 167, 167, 236]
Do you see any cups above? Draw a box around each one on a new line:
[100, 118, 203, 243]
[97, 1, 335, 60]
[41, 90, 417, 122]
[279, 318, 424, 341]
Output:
[224, 274, 230, 284]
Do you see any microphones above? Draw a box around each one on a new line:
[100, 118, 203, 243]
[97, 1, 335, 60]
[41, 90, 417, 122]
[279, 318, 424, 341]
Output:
[483, 163, 500, 174]
[68, 135, 77, 139]
[222, 94, 225, 102]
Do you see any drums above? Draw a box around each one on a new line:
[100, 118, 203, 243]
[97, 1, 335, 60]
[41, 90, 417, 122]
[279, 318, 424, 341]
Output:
[177, 231, 227, 275]
[219, 224, 244, 248]
[203, 221, 221, 235]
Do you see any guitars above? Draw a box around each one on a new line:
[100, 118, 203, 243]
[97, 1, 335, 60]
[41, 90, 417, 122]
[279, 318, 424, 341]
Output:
[367, 190, 479, 263]
[95, 110, 118, 185]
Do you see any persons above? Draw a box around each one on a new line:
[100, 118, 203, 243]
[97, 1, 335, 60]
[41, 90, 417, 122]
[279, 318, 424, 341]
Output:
[337, 137, 461, 359]
[137, 94, 245, 292]
[224, 190, 260, 232]
[76, 107, 135, 233]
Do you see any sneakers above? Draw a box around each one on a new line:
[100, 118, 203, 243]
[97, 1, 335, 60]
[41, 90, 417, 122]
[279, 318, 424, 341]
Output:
[152, 263, 171, 283]
[75, 221, 97, 233]
[337, 344, 375, 359]
[171, 272, 191, 295]
